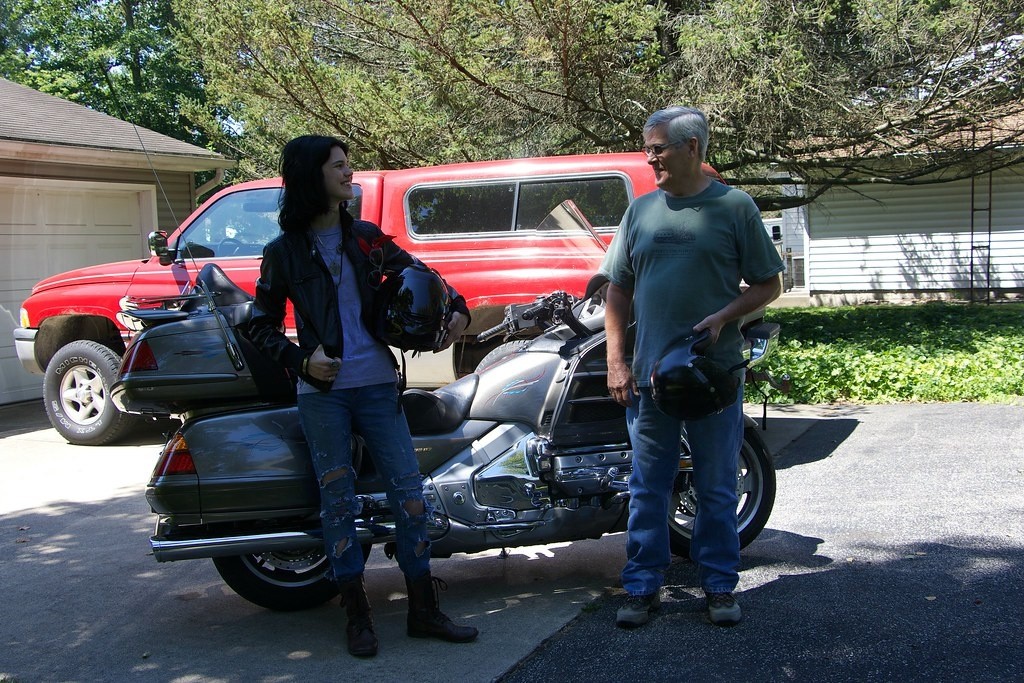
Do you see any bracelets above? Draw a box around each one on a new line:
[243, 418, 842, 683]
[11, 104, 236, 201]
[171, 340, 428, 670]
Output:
[303, 357, 307, 375]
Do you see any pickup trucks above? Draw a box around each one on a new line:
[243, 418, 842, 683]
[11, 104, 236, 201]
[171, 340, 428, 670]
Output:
[13, 149, 728, 448]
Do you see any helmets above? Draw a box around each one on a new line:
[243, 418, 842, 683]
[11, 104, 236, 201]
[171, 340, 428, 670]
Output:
[366, 266, 453, 352]
[650, 328, 741, 420]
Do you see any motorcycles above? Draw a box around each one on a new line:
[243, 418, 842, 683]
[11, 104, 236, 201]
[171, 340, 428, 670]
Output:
[107, 201, 778, 611]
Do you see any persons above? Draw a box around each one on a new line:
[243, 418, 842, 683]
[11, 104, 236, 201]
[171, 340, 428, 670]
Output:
[247, 135, 480, 656]
[596, 106, 788, 627]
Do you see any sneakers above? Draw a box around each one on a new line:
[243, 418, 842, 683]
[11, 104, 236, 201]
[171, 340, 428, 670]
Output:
[616, 592, 660, 624]
[709, 593, 741, 623]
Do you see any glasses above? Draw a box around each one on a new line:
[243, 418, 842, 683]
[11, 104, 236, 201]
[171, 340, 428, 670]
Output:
[642, 141, 679, 156]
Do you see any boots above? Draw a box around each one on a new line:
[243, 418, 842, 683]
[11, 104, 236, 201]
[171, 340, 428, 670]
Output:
[336, 575, 378, 655]
[404, 570, 479, 643]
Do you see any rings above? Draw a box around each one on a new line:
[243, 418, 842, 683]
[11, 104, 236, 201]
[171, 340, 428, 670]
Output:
[327, 377, 331, 382]
[331, 358, 340, 367]
